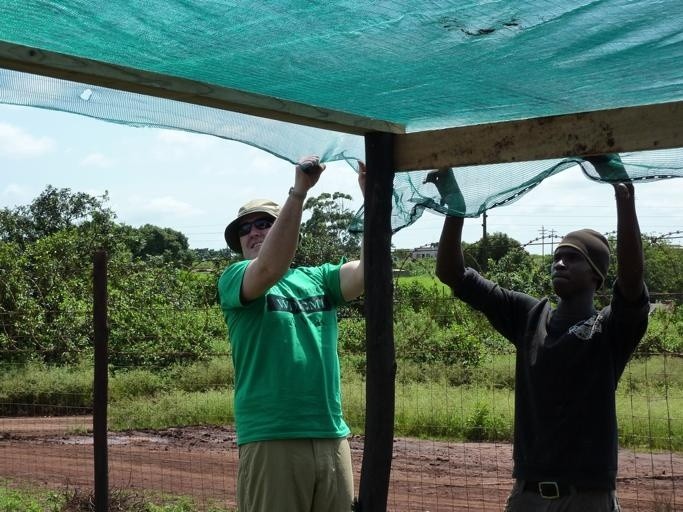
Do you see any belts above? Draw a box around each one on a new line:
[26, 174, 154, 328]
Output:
[521, 480, 600, 500]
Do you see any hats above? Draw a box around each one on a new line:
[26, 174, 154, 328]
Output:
[555, 228, 612, 286]
[222, 197, 303, 254]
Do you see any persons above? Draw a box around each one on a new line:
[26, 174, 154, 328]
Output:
[216, 155, 366, 512]
[422, 152, 651, 512]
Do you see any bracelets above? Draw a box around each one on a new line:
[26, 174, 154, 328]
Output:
[287, 186, 308, 201]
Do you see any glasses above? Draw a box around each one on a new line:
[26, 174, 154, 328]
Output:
[234, 215, 275, 239]
